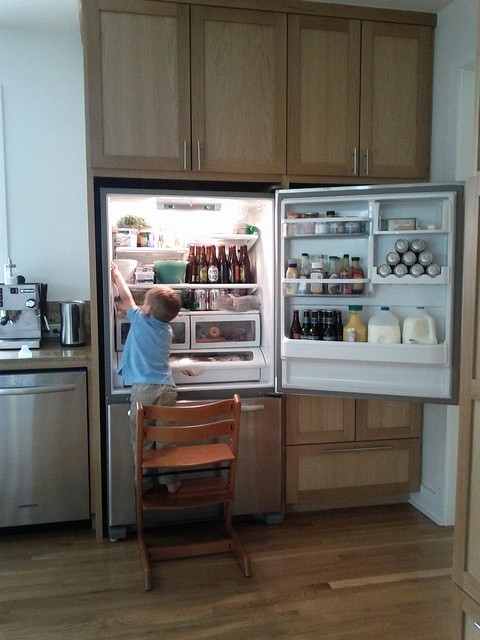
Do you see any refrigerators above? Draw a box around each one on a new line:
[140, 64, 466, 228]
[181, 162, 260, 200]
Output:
[99, 180, 465, 547]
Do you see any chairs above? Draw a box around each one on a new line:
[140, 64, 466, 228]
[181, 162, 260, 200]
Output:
[133, 393, 251, 592]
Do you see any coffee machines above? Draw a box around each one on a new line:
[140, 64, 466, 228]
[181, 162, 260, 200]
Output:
[0, 281, 48, 350]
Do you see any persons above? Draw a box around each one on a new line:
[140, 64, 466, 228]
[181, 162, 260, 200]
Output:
[111, 261, 182, 495]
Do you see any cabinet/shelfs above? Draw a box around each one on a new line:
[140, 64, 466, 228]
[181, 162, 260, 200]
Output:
[88, 2, 288, 175]
[288, 7, 434, 182]
[282, 390, 419, 510]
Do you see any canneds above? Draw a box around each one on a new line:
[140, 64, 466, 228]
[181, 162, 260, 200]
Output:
[287, 211, 300, 235]
[194, 289, 207, 311]
[393, 264, 408, 278]
[418, 251, 433, 267]
[401, 250, 417, 267]
[427, 263, 441, 278]
[303, 212, 318, 233]
[315, 219, 328, 234]
[410, 239, 426, 253]
[377, 263, 392, 278]
[410, 263, 425, 278]
[345, 216, 361, 233]
[208, 289, 220, 311]
[386, 252, 401, 266]
[394, 239, 409, 254]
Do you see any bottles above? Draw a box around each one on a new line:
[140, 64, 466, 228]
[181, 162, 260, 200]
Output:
[318, 309, 322, 325]
[186, 245, 194, 282]
[229, 246, 240, 283]
[290, 310, 301, 338]
[326, 256, 339, 293]
[285, 259, 298, 293]
[318, 309, 326, 340]
[228, 246, 232, 261]
[239, 246, 243, 263]
[199, 246, 208, 283]
[351, 257, 364, 293]
[298, 253, 311, 294]
[336, 310, 343, 340]
[240, 245, 250, 283]
[307, 310, 318, 339]
[339, 254, 351, 293]
[310, 261, 325, 293]
[300, 309, 310, 339]
[219, 246, 229, 283]
[192, 246, 200, 282]
[206, 246, 211, 262]
[318, 254, 324, 264]
[208, 245, 219, 283]
[323, 311, 336, 340]
[218, 247, 222, 262]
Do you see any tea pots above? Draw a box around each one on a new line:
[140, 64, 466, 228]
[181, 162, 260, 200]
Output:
[59, 299, 87, 347]
